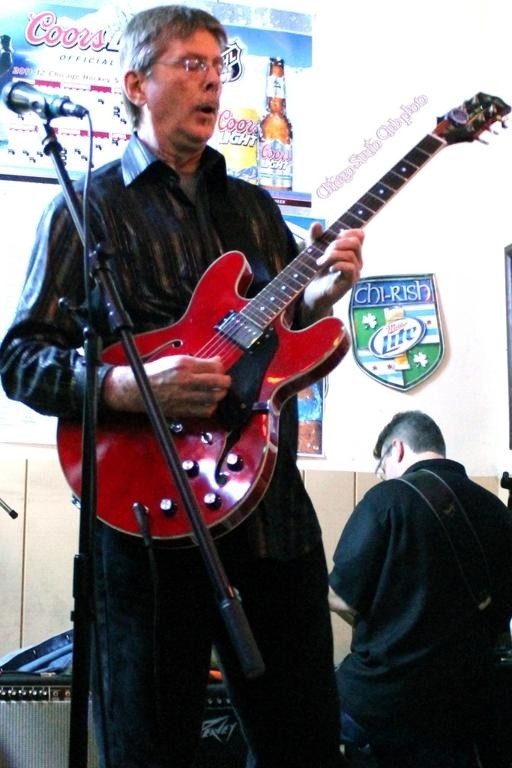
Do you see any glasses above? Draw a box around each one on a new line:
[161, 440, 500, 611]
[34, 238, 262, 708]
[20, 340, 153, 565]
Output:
[374, 445, 393, 481]
[150, 56, 233, 84]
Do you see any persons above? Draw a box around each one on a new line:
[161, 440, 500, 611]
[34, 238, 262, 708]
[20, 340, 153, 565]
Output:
[0, 4, 367, 767]
[326, 409, 509, 768]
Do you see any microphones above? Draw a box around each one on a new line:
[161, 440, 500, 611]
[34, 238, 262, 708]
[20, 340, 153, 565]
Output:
[1, 79, 89, 118]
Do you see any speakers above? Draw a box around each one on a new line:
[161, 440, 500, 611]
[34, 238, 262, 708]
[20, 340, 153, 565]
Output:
[0, 671, 102, 768]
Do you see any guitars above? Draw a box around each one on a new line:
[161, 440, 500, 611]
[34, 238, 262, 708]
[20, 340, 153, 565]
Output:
[57, 92, 512, 546]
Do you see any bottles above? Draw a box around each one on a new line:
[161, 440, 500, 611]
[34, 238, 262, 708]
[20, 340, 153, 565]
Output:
[251, 54, 299, 192]
